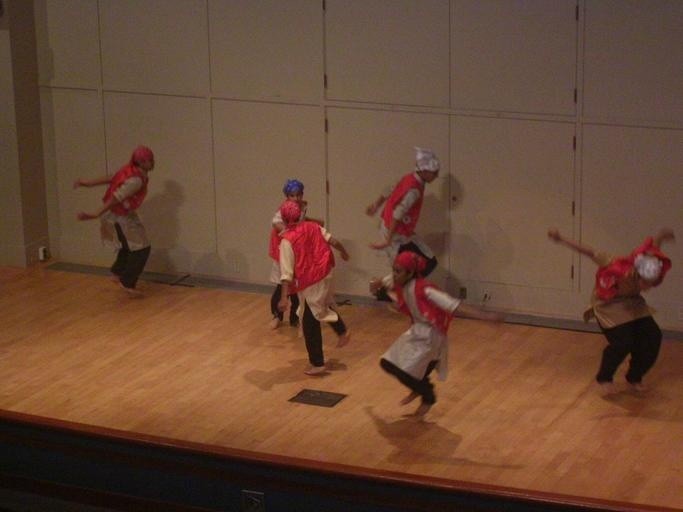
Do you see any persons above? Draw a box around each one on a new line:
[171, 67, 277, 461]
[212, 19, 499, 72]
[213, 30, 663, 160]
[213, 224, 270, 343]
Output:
[366, 152, 436, 306]
[74, 146, 155, 298]
[368, 252, 494, 416]
[278, 201, 350, 376]
[545, 228, 675, 393]
[270, 180, 308, 328]
